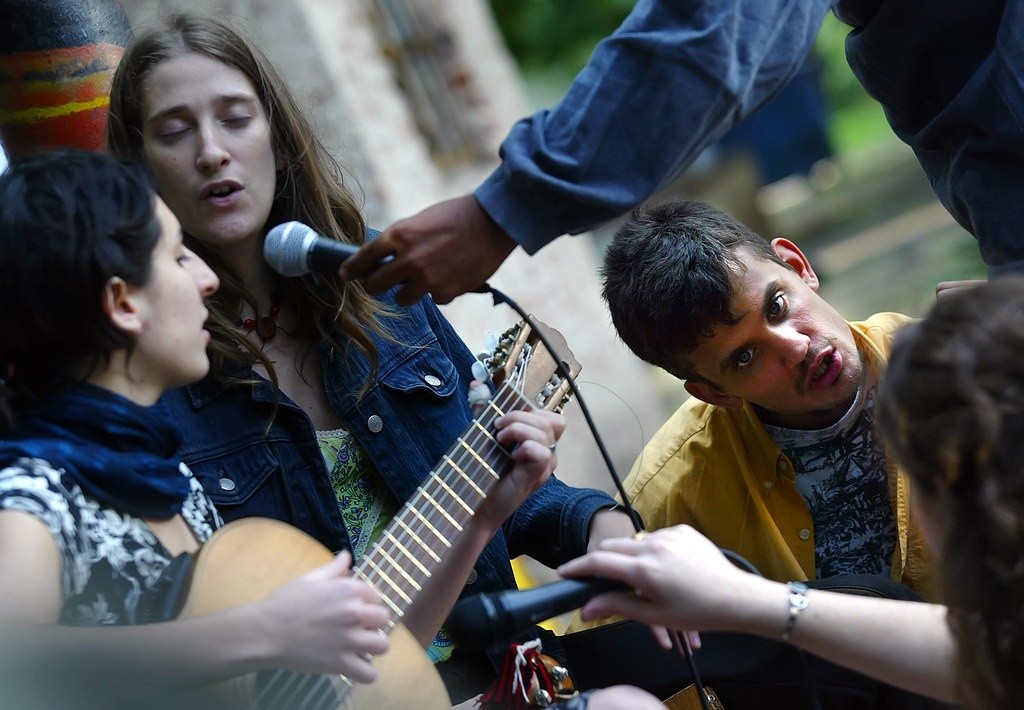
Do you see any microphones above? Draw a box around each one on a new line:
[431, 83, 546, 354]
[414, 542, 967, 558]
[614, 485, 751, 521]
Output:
[453, 575, 634, 646]
[264, 221, 489, 293]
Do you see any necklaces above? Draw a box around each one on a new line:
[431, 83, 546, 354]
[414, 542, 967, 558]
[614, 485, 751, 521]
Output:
[226, 290, 284, 341]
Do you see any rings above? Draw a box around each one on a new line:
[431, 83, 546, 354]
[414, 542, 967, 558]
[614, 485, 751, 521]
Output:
[633, 530, 647, 540]
[548, 443, 558, 450]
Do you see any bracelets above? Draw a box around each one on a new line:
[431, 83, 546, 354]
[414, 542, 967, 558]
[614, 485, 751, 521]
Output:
[781, 581, 808, 640]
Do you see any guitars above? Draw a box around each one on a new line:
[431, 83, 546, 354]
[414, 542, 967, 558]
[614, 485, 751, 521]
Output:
[93, 314, 586, 710]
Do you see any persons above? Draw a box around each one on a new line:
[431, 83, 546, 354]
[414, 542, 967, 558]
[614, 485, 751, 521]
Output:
[559, 199, 1024, 710]
[338, 0, 1024, 306]
[0, 147, 565, 710]
[104, 15, 701, 706]
